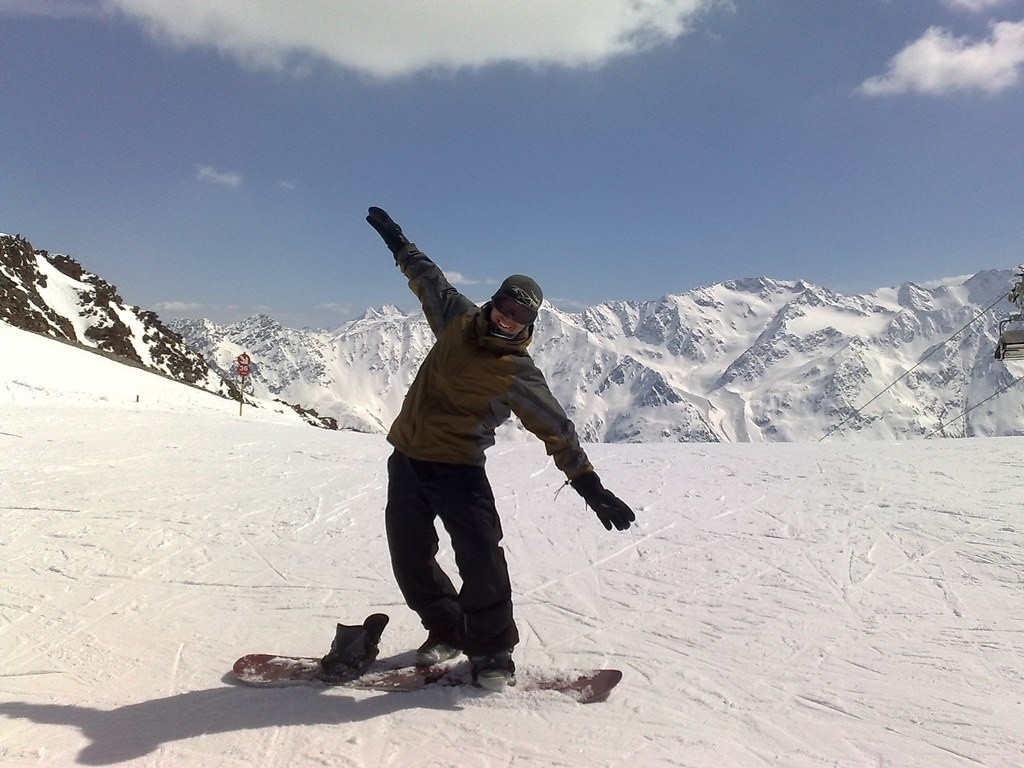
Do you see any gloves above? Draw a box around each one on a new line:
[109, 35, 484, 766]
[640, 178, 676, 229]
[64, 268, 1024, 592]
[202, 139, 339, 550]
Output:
[571, 470, 635, 531]
[366, 206, 409, 257]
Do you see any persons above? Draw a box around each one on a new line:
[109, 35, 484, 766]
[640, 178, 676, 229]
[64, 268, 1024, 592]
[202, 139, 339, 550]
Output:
[365, 205, 637, 693]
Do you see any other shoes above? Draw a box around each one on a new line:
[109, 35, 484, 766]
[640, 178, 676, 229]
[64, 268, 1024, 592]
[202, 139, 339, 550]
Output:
[412, 635, 460, 667]
[473, 649, 512, 691]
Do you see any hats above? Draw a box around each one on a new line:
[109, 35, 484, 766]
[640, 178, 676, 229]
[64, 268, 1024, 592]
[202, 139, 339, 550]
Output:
[498, 274, 543, 311]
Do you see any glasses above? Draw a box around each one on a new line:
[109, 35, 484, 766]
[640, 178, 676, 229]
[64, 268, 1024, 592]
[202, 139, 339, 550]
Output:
[492, 291, 538, 325]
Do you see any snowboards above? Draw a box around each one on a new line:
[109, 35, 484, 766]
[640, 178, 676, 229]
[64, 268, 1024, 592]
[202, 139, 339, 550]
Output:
[232, 653, 622, 704]
